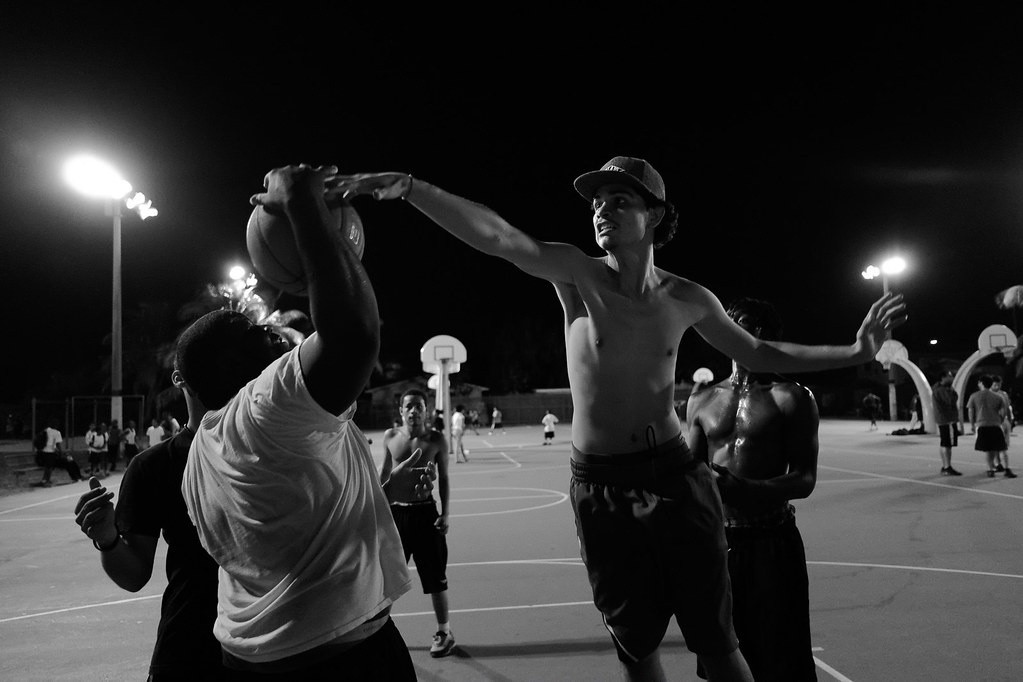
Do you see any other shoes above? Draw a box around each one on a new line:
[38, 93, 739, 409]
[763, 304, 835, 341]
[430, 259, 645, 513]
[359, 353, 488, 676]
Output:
[1004, 468, 1017, 478]
[987, 470, 993, 477]
[941, 466, 961, 477]
[995, 464, 1004, 473]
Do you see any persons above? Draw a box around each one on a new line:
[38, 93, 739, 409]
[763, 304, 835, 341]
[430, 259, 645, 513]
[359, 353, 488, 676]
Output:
[73, 303, 219, 682]
[392, 417, 401, 428]
[32, 409, 180, 487]
[863, 393, 881, 425]
[325, 157, 908, 682]
[932, 368, 962, 476]
[426, 405, 502, 463]
[684, 297, 819, 682]
[177, 163, 418, 682]
[541, 410, 559, 446]
[380, 389, 456, 658]
[967, 374, 1018, 478]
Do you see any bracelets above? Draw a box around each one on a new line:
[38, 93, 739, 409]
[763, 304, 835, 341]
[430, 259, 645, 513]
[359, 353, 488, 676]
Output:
[93, 523, 121, 552]
[401, 174, 413, 201]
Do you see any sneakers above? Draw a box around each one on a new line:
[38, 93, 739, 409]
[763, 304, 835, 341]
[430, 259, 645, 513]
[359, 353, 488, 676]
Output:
[430, 626, 456, 656]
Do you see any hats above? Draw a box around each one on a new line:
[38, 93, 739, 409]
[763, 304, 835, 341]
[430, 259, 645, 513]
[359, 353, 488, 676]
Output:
[573, 155, 666, 210]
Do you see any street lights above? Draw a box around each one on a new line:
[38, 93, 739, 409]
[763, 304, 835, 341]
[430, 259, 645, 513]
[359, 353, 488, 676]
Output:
[65, 153, 123, 456]
[880, 256, 908, 419]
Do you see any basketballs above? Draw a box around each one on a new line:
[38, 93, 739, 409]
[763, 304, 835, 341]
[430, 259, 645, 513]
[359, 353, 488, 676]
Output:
[244, 192, 365, 299]
